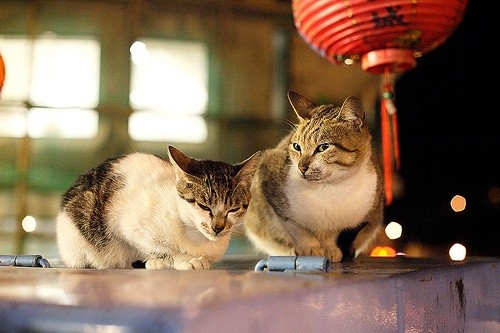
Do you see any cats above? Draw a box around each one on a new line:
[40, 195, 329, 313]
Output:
[240, 91, 384, 264]
[54, 145, 262, 271]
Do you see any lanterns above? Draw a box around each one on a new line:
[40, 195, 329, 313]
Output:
[291, 0, 467, 204]
